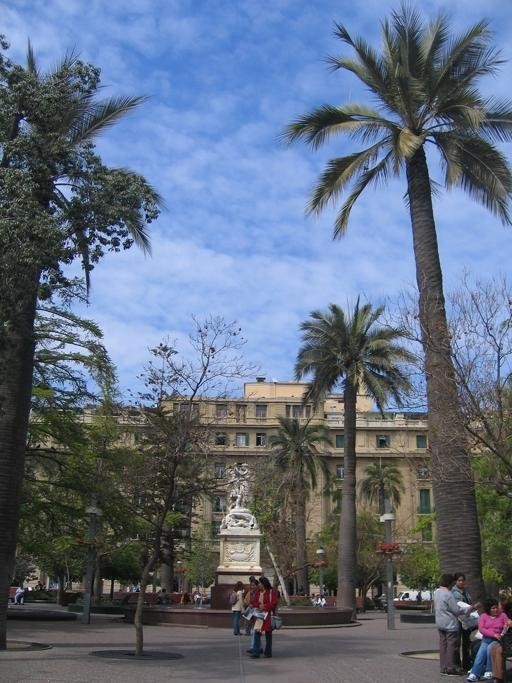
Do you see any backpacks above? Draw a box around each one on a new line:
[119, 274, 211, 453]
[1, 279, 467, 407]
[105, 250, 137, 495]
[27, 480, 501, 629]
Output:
[230, 591, 239, 604]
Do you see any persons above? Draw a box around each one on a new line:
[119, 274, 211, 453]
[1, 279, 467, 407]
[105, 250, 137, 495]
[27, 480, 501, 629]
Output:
[310, 593, 328, 608]
[416, 592, 422, 605]
[226, 463, 251, 513]
[230, 575, 278, 659]
[433, 572, 512, 683]
[35, 581, 43, 591]
[13, 584, 25, 605]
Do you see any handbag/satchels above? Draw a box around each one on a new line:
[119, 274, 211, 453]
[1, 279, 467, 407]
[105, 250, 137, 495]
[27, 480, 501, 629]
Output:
[271, 615, 281, 630]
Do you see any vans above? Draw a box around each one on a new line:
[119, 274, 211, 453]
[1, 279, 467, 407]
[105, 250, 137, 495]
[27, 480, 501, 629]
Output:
[393, 589, 431, 602]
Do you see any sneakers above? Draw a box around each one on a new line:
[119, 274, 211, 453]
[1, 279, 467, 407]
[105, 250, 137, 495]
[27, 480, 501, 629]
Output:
[439, 672, 463, 677]
[246, 649, 272, 658]
[483, 672, 491, 679]
[467, 674, 479, 683]
